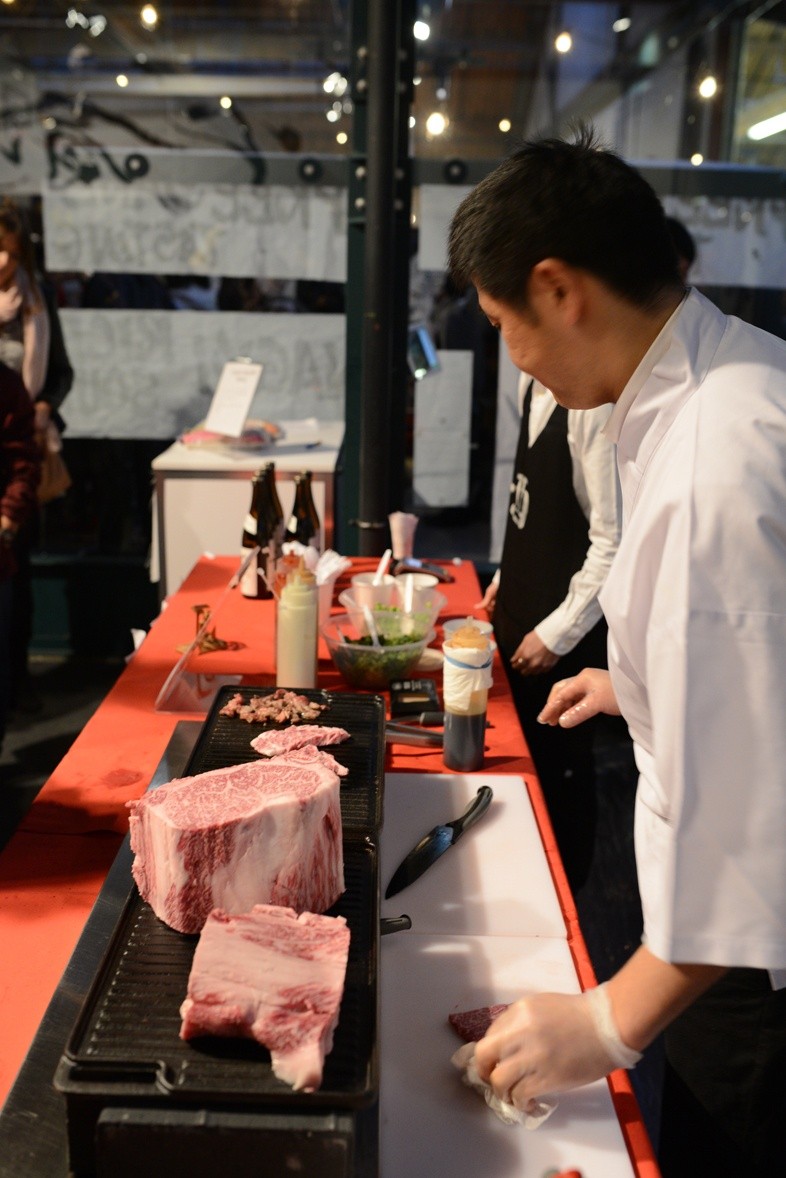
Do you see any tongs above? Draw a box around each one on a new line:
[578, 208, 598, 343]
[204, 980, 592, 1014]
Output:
[383, 722, 490, 753]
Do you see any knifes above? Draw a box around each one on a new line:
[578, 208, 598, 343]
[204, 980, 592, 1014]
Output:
[384, 785, 494, 898]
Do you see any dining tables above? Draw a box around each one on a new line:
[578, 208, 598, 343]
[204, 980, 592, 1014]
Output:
[0, 553, 663, 1178]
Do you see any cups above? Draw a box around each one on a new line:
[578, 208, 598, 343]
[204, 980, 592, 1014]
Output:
[396, 573, 438, 606]
[352, 571, 396, 605]
[388, 511, 420, 574]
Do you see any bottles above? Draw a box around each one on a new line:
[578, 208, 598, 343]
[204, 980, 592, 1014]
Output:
[273, 547, 320, 690]
[283, 469, 322, 557]
[238, 460, 283, 599]
[443, 615, 497, 774]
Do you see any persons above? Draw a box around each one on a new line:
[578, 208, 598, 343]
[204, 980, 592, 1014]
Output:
[449, 117, 785, 1116]
[0, 197, 72, 696]
[0, 360, 41, 748]
[475, 370, 625, 860]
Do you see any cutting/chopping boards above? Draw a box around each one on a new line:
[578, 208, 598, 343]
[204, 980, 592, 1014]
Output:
[381, 769, 638, 1178]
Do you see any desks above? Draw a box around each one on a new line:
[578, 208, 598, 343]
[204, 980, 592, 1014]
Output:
[147, 418, 344, 618]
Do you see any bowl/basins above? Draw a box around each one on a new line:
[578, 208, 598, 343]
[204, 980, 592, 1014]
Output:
[319, 610, 438, 692]
[338, 584, 446, 636]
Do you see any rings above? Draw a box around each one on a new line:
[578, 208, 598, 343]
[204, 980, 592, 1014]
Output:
[518, 658, 524, 665]
